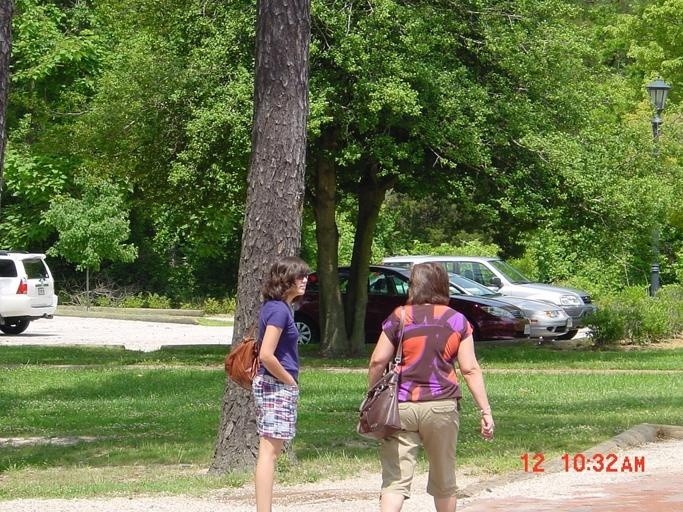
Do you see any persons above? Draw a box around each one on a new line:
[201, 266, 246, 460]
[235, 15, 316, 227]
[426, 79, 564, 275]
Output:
[249, 256, 312, 512]
[366, 260, 497, 512]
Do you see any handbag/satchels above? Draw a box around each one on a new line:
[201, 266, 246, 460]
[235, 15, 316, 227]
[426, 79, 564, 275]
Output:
[225, 336, 260, 392]
[357, 366, 402, 440]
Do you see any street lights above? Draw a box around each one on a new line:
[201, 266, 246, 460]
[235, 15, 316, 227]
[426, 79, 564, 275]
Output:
[644, 78, 671, 297]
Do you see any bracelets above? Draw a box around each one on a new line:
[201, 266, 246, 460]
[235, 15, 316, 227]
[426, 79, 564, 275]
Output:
[479, 405, 492, 415]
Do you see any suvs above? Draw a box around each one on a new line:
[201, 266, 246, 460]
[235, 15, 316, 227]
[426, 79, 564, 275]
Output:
[0, 250, 59, 336]
